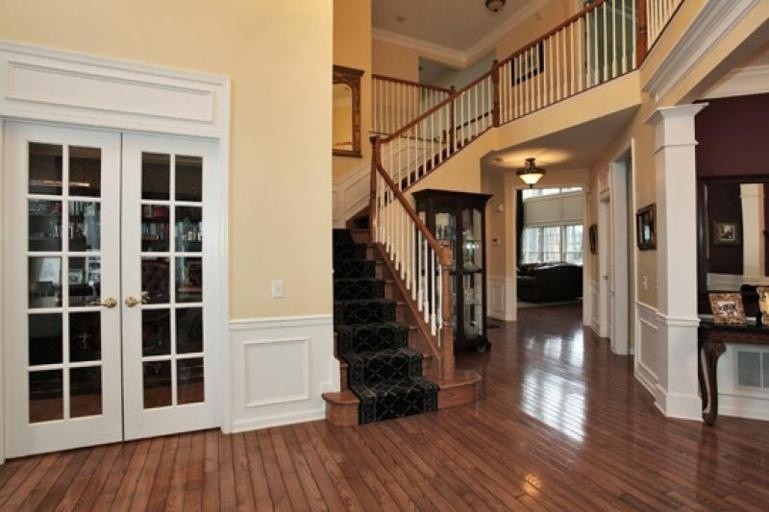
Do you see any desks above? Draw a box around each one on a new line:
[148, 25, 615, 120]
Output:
[699, 322, 769, 427]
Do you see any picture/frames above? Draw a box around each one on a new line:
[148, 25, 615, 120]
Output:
[636, 201, 657, 250]
[510, 41, 544, 86]
[588, 224, 598, 254]
[707, 220, 769, 330]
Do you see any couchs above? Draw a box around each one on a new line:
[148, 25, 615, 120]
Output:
[518, 263, 584, 304]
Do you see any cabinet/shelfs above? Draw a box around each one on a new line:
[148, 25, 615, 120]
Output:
[413, 187, 491, 356]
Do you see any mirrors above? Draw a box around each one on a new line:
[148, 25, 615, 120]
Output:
[330, 63, 366, 157]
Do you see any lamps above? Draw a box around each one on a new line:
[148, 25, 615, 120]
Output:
[486, 1, 507, 14]
[516, 158, 545, 188]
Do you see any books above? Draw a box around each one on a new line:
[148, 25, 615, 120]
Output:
[46, 199, 201, 242]
